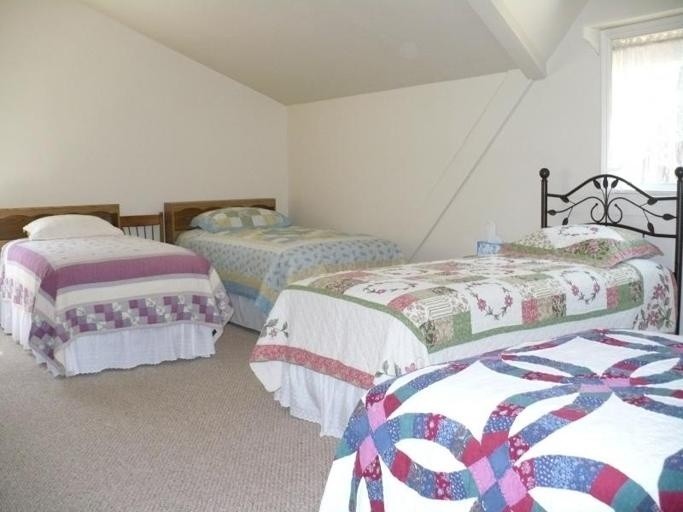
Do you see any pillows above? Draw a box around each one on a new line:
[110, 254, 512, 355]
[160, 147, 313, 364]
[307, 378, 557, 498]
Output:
[500, 222, 666, 269]
[18, 212, 125, 241]
[190, 204, 293, 233]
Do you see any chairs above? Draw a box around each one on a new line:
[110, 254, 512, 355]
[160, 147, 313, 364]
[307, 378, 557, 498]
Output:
[118, 211, 172, 246]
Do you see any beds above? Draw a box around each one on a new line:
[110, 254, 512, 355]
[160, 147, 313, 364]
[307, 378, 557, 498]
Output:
[247, 164, 682, 442]
[0, 202, 234, 382]
[163, 196, 405, 336]
[313, 322, 683, 512]
